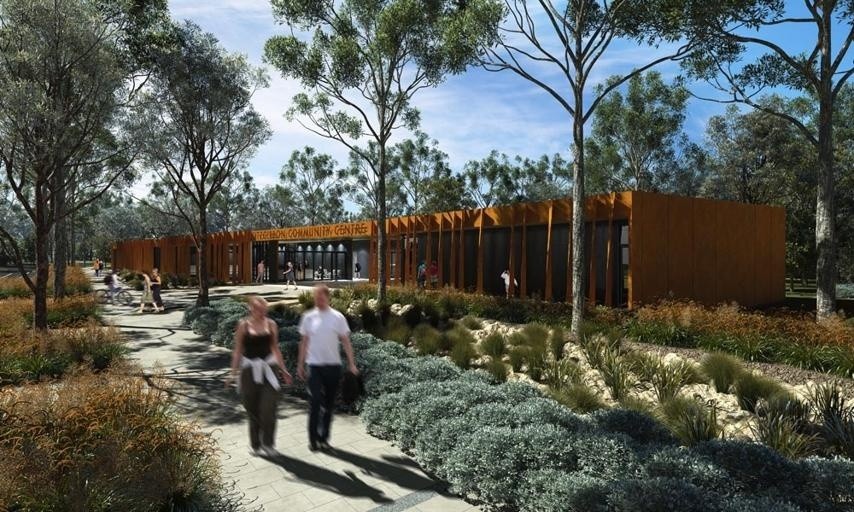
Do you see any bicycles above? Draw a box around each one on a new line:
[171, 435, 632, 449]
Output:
[93, 288, 134, 306]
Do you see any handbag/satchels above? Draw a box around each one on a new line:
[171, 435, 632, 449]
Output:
[341, 370, 364, 405]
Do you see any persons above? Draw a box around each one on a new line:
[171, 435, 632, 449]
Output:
[304, 259, 361, 278]
[256, 259, 266, 284]
[418, 259, 429, 287]
[428, 260, 439, 288]
[230, 296, 293, 456]
[94, 258, 164, 313]
[296, 284, 361, 451]
[283, 261, 298, 291]
[501, 268, 518, 299]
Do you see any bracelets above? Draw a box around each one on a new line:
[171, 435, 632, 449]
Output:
[281, 367, 287, 371]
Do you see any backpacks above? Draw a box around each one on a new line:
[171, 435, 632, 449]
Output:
[103, 274, 113, 286]
